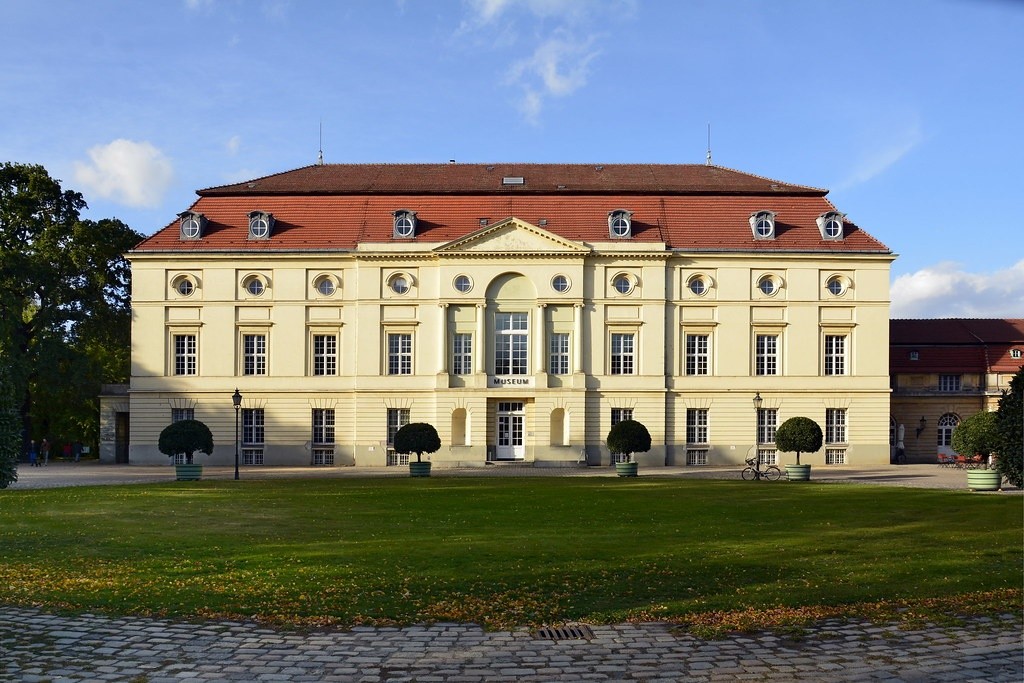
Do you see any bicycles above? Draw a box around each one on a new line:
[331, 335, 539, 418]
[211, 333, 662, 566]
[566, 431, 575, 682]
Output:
[742, 457, 781, 482]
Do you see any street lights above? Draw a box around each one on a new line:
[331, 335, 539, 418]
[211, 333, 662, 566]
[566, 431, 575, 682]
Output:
[231, 388, 243, 480]
[752, 391, 763, 481]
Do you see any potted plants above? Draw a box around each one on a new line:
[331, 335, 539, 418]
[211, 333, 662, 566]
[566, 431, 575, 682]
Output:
[392, 422, 442, 478]
[951, 409, 1003, 490]
[606, 419, 653, 478]
[774, 416, 824, 481]
[157, 419, 215, 482]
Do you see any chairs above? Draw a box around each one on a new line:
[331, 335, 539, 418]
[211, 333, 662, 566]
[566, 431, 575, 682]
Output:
[936, 453, 982, 469]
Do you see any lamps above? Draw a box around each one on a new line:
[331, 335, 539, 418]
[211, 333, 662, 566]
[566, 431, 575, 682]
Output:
[916, 414, 927, 440]
